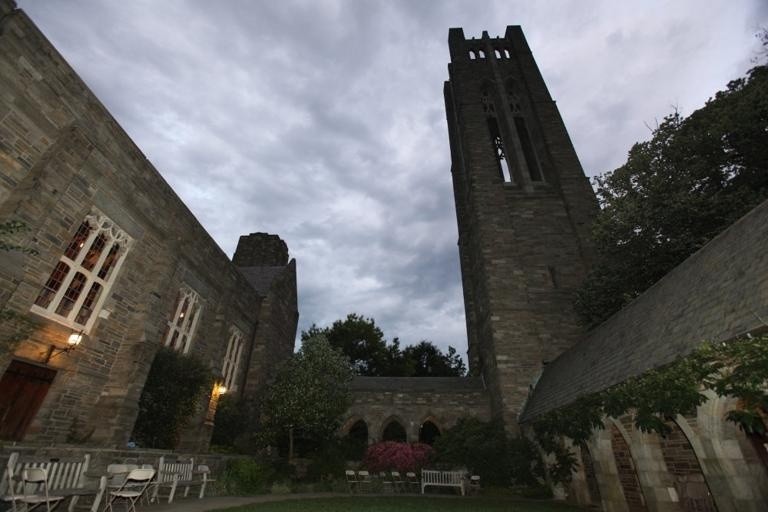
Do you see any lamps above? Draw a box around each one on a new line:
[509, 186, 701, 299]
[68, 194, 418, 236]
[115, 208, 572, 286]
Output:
[43, 329, 84, 364]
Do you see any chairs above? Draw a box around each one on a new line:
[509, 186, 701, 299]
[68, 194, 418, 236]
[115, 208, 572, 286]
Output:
[0, 451, 217, 512]
[344, 470, 420, 496]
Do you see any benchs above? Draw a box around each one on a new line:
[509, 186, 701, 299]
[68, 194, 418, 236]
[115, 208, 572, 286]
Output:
[420, 467, 466, 496]
